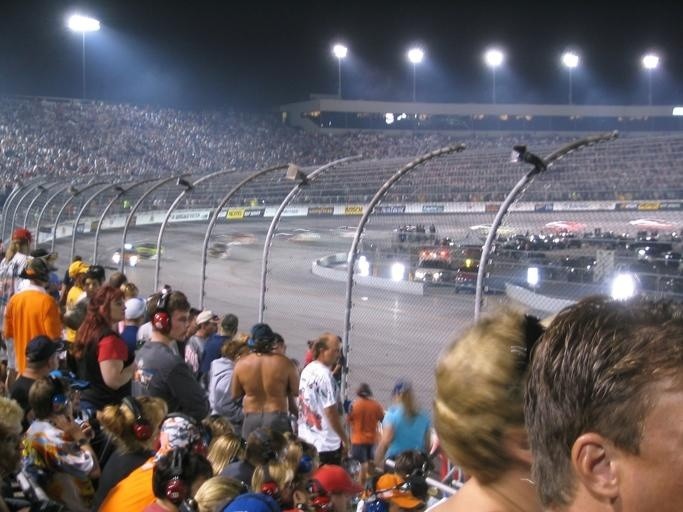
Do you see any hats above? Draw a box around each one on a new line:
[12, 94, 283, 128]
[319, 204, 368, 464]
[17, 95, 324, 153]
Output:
[196, 310, 221, 325]
[390, 381, 410, 396]
[123, 296, 148, 320]
[26, 335, 62, 361]
[69, 261, 91, 278]
[376, 474, 426, 509]
[84, 265, 105, 279]
[355, 382, 373, 399]
[21, 263, 58, 279]
[11, 228, 33, 242]
[31, 248, 53, 260]
[311, 463, 365, 497]
[160, 413, 210, 449]
[46, 368, 90, 392]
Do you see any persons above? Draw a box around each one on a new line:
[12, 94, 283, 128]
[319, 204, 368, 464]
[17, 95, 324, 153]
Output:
[0, 95, 682, 510]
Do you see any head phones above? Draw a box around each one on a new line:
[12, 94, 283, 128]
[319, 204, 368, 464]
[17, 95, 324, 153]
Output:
[260, 449, 281, 495]
[48, 372, 67, 414]
[404, 451, 429, 484]
[123, 395, 153, 441]
[151, 411, 208, 456]
[246, 338, 257, 350]
[164, 445, 190, 503]
[363, 472, 389, 512]
[152, 285, 172, 334]
[20, 267, 50, 282]
[305, 477, 334, 512]
[293, 440, 313, 473]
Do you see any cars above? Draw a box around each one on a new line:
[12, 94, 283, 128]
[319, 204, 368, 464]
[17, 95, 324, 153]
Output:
[109, 223, 368, 270]
[390, 217, 682, 299]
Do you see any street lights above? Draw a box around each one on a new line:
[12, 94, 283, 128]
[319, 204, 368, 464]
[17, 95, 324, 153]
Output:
[331, 44, 348, 97]
[640, 52, 661, 106]
[482, 46, 504, 103]
[408, 49, 426, 102]
[561, 49, 581, 105]
[69, 15, 101, 102]
